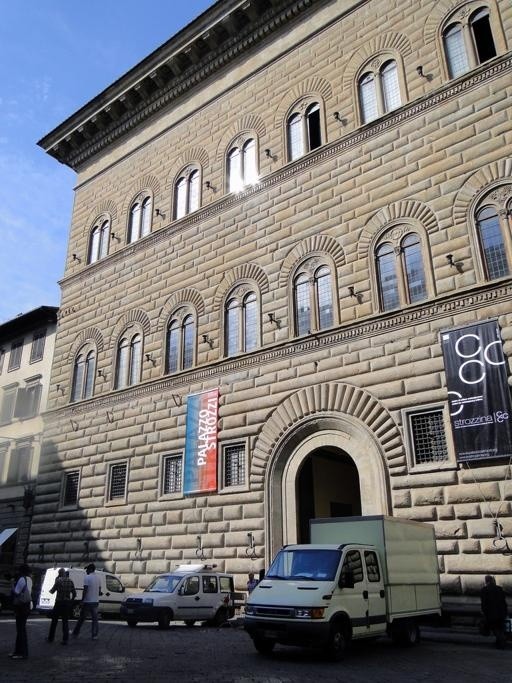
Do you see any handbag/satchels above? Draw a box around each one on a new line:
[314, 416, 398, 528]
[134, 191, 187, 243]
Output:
[12, 590, 32, 607]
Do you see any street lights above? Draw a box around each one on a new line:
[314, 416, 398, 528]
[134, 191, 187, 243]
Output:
[22, 488, 34, 562]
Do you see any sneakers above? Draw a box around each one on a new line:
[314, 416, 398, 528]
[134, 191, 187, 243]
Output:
[8, 652, 28, 659]
[45, 636, 69, 645]
[68, 629, 98, 640]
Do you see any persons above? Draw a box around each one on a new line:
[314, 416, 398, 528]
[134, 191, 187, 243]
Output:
[8, 563, 102, 659]
[247, 573, 260, 595]
[479, 576, 507, 650]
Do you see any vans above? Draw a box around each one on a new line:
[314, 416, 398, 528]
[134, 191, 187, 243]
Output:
[36, 565, 235, 626]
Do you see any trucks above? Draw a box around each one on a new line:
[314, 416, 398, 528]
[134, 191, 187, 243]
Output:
[243, 515, 443, 659]
